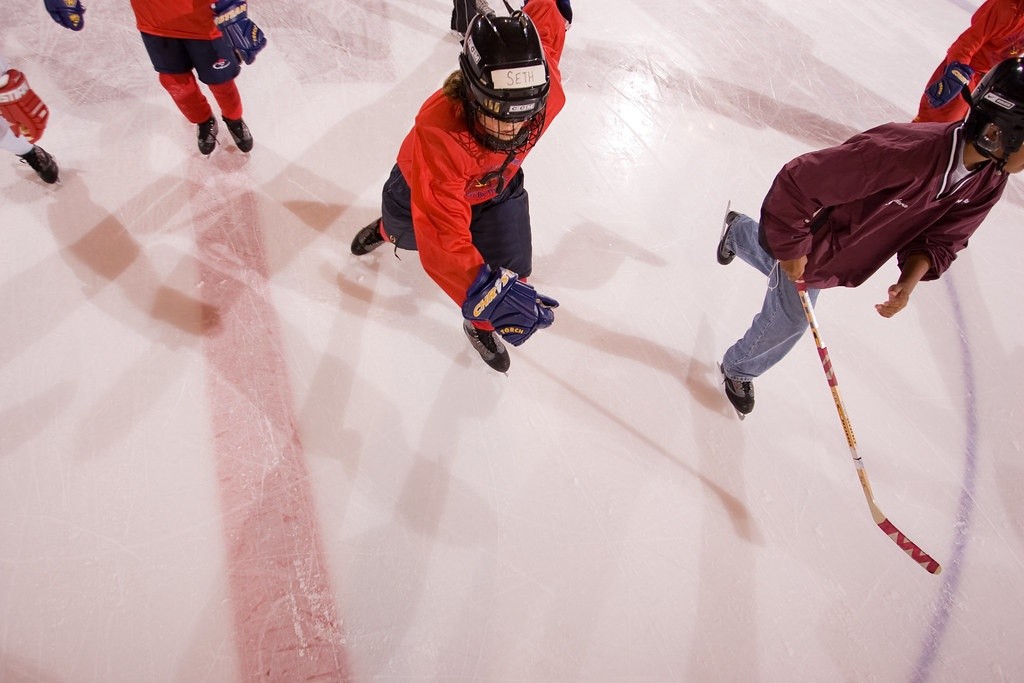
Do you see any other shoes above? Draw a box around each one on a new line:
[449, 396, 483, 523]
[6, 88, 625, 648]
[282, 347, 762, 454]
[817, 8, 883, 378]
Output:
[463, 320, 510, 378]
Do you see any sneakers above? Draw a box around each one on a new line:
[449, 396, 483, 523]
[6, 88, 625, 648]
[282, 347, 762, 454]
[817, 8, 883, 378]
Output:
[717, 200, 740, 265]
[351, 217, 384, 256]
[718, 362, 756, 420]
[17, 145, 63, 187]
[222, 116, 254, 156]
[198, 116, 219, 159]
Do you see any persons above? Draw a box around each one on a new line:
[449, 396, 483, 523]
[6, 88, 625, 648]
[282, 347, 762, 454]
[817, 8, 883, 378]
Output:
[0, 56, 64, 185]
[912, 0, 1024, 123]
[42, 0, 267, 160]
[350, 1, 572, 380]
[716, 56, 1024, 422]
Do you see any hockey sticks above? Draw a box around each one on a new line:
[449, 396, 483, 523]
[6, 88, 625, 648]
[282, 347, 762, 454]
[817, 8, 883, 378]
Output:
[944, 66, 971, 107]
[502, 0, 515, 14]
[793, 275, 943, 576]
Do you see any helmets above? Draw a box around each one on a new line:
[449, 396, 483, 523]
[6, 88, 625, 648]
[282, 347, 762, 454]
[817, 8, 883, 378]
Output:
[459, 10, 551, 157]
[960, 56, 1024, 152]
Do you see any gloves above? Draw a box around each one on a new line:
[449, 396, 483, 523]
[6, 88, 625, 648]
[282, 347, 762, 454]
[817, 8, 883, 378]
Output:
[211, 0, 267, 66]
[461, 263, 560, 348]
[925, 63, 974, 108]
[44, 0, 85, 31]
[0, 70, 49, 144]
